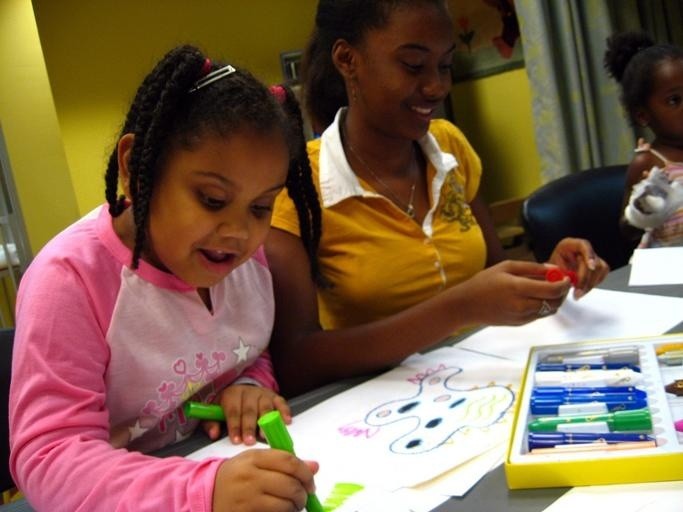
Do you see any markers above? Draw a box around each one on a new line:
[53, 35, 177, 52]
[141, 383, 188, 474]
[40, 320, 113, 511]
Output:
[257, 410, 325, 512]
[185, 400, 226, 421]
[528, 342, 683, 454]
[545, 267, 576, 287]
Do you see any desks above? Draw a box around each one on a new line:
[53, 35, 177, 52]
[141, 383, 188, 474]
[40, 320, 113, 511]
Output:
[1, 266, 682, 512]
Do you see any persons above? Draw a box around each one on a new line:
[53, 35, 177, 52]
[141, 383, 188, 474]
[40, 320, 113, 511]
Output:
[8, 43, 336, 512]
[263, 1, 611, 398]
[604, 29, 682, 245]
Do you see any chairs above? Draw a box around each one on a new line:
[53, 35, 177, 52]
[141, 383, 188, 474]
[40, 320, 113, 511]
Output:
[521, 165, 645, 278]
[0, 329, 17, 507]
[470, 191, 528, 271]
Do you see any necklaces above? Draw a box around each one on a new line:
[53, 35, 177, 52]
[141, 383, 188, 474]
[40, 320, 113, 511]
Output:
[340, 123, 418, 218]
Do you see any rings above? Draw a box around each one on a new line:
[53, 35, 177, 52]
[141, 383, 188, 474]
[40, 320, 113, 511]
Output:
[538, 300, 552, 317]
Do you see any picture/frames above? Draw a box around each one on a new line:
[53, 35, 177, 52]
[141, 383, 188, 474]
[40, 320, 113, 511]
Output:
[439, 0, 526, 85]
